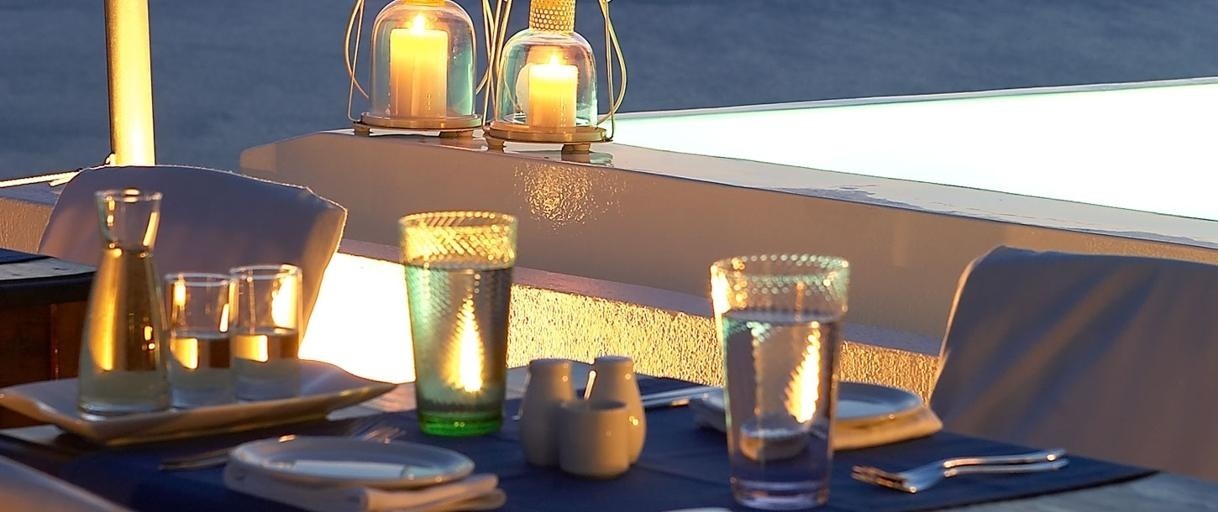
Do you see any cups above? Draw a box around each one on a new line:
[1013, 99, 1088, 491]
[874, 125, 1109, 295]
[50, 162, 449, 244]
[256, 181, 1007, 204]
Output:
[556, 400, 632, 479]
[709, 252, 855, 512]
[157, 263, 304, 412]
[395, 205, 521, 440]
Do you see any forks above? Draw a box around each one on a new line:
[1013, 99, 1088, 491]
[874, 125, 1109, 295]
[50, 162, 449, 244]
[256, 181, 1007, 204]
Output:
[851, 448, 1070, 493]
[159, 411, 397, 474]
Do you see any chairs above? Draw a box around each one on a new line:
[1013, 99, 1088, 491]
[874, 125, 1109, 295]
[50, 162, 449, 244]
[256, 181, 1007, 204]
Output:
[40, 166, 347, 339]
[928, 247, 1218, 479]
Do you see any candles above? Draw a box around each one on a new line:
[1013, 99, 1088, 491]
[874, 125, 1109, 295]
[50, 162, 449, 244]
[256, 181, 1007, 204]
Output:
[389, 18, 448, 118]
[527, 54, 578, 130]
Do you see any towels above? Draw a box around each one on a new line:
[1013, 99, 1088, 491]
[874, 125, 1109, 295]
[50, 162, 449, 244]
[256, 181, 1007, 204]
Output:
[220, 458, 510, 512]
[696, 399, 943, 450]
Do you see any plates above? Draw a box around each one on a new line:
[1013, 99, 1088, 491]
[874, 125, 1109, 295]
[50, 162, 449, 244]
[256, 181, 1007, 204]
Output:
[228, 436, 476, 492]
[0, 358, 394, 451]
[702, 380, 931, 429]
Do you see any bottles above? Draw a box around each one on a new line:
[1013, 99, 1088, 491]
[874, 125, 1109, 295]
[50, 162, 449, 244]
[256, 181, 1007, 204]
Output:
[523, 357, 647, 467]
[77, 188, 169, 416]
